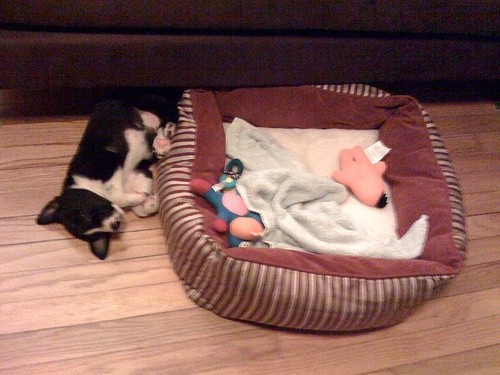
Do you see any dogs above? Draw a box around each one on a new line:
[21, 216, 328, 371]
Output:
[34, 92, 177, 260]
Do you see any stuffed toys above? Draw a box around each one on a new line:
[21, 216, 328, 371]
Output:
[191, 174, 266, 247]
[334, 146, 387, 208]
